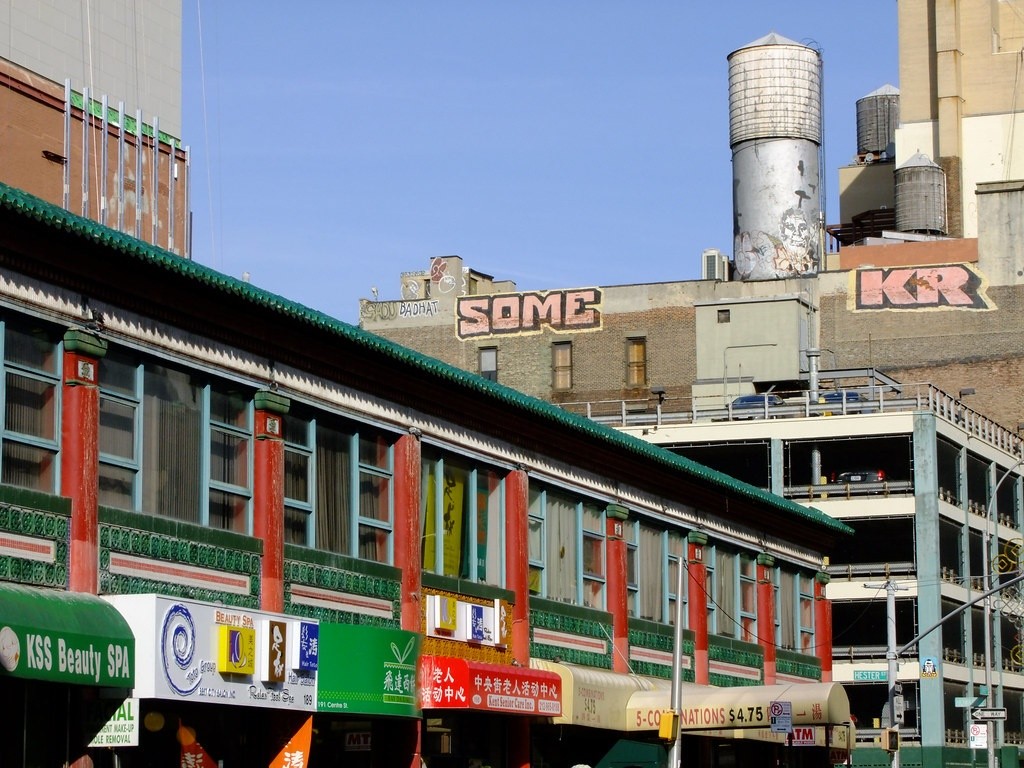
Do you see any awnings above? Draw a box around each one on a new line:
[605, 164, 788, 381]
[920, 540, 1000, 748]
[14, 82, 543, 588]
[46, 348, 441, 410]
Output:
[0, 581, 136, 689]
[420, 655, 562, 716]
[530, 658, 856, 768]
